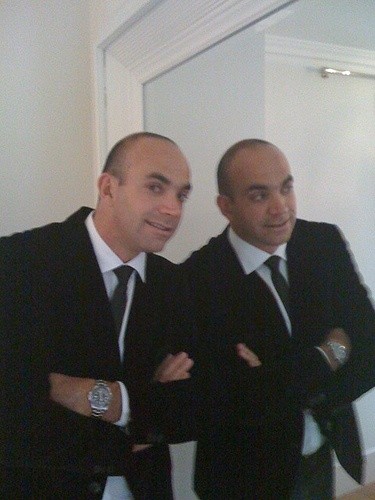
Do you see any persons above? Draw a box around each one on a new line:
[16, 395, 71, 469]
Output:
[177, 138, 375, 498]
[0, 130, 234, 498]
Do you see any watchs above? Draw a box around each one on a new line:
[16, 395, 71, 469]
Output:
[322, 338, 351, 366]
[87, 376, 113, 417]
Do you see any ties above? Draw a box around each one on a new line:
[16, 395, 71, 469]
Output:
[110, 266, 135, 344]
[264, 256, 294, 329]
